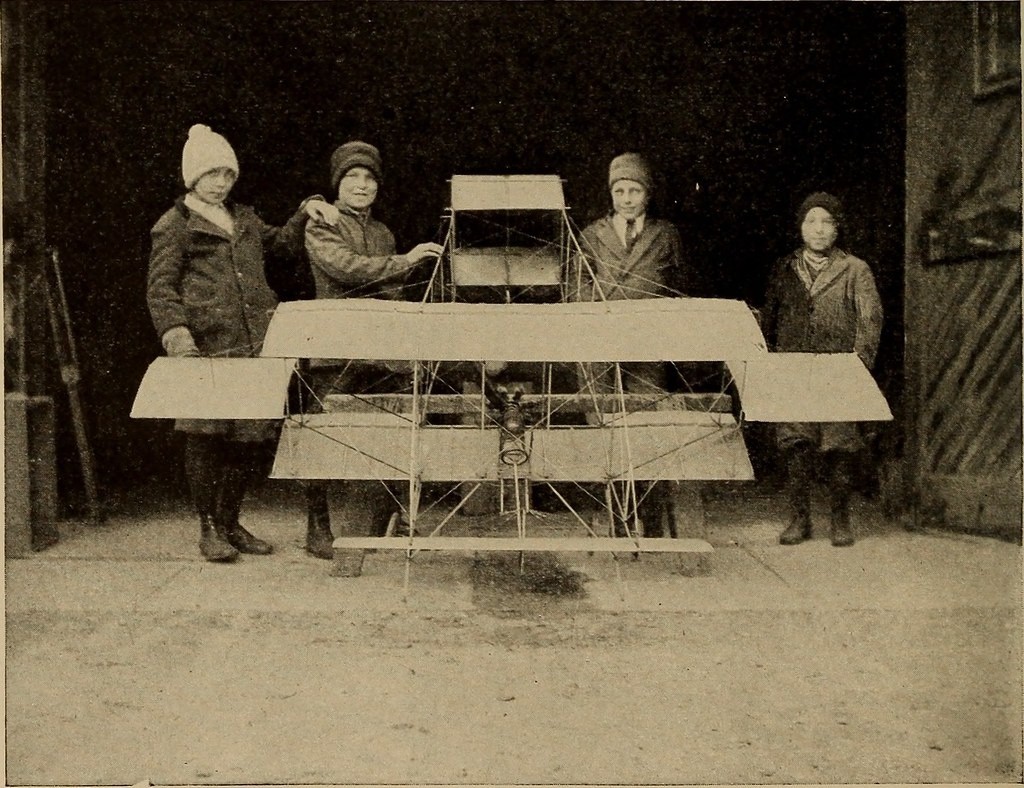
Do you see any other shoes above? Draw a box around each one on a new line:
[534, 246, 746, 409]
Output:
[199, 513, 273, 560]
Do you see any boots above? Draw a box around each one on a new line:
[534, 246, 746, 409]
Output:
[830, 490, 854, 547]
[780, 487, 813, 544]
[374, 484, 419, 537]
[306, 487, 335, 558]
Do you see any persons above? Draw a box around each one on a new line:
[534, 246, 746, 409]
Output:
[760, 191, 884, 547]
[569, 151, 697, 539]
[146, 124, 342, 562]
[305, 141, 445, 560]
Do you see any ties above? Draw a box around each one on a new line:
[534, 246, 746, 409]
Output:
[625, 220, 634, 248]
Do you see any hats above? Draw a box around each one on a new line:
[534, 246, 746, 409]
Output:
[182, 125, 239, 186]
[798, 192, 844, 236]
[331, 141, 384, 191]
[610, 153, 654, 192]
